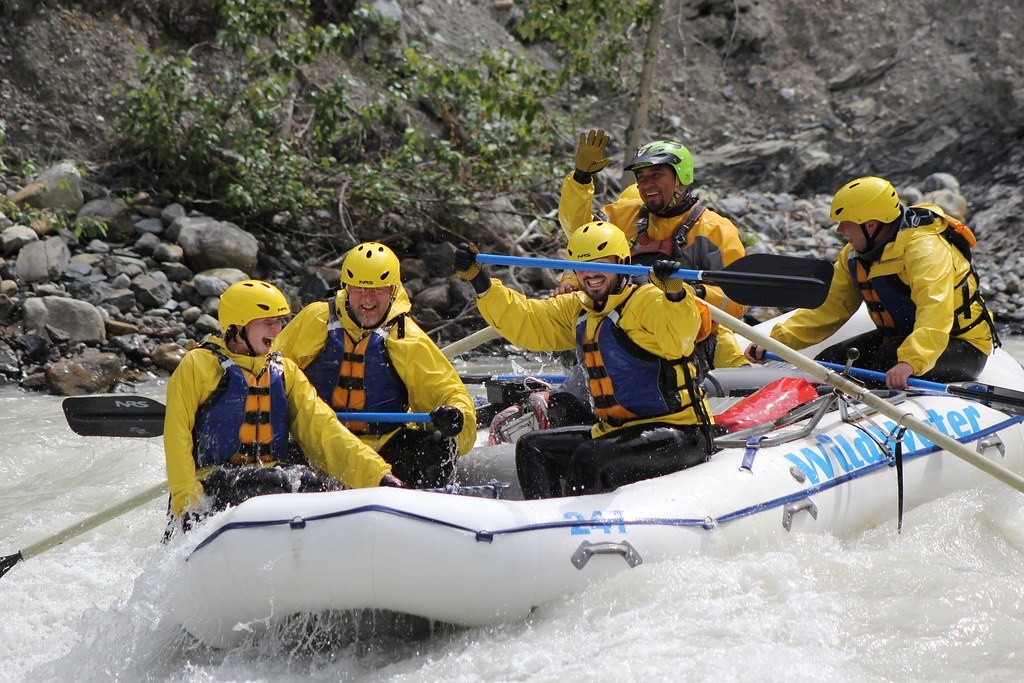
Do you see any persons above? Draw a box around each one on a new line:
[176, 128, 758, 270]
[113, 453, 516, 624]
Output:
[269, 243, 476, 488]
[162, 280, 400, 534]
[548, 183, 750, 367]
[454, 222, 716, 498]
[559, 128, 750, 319]
[743, 175, 992, 389]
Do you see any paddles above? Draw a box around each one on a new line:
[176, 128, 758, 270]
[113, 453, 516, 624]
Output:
[692, 296, 1024, 493]
[463, 245, 836, 309]
[62, 394, 462, 441]
[0, 323, 497, 581]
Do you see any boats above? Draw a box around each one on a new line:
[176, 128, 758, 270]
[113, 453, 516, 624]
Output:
[142, 299, 1024, 662]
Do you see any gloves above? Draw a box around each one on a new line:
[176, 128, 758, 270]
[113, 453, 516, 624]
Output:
[648, 260, 686, 302]
[683, 278, 705, 300]
[429, 405, 464, 436]
[454, 242, 492, 294]
[573, 128, 613, 184]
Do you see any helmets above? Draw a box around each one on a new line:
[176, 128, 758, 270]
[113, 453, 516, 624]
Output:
[829, 177, 901, 224]
[340, 242, 400, 298]
[218, 280, 290, 334]
[623, 141, 694, 186]
[567, 221, 631, 285]
